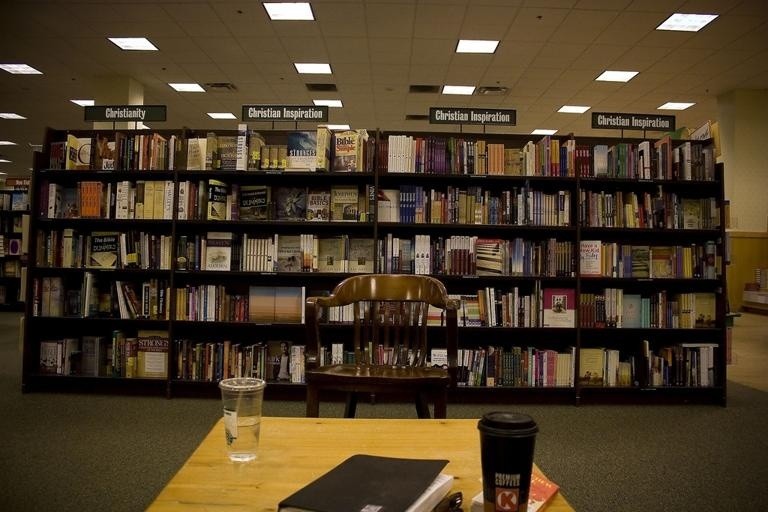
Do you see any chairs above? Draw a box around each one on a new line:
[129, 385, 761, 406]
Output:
[305, 274, 458, 418]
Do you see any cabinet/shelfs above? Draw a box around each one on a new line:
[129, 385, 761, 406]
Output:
[0, 127, 727, 408]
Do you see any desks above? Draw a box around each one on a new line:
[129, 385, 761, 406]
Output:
[145, 416, 575, 512]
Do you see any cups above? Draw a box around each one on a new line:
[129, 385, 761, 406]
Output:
[220, 378, 266, 462]
[477, 411, 538, 511]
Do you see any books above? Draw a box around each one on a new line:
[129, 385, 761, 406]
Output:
[2, 120, 728, 391]
[278, 452, 454, 512]
[469, 471, 560, 512]
